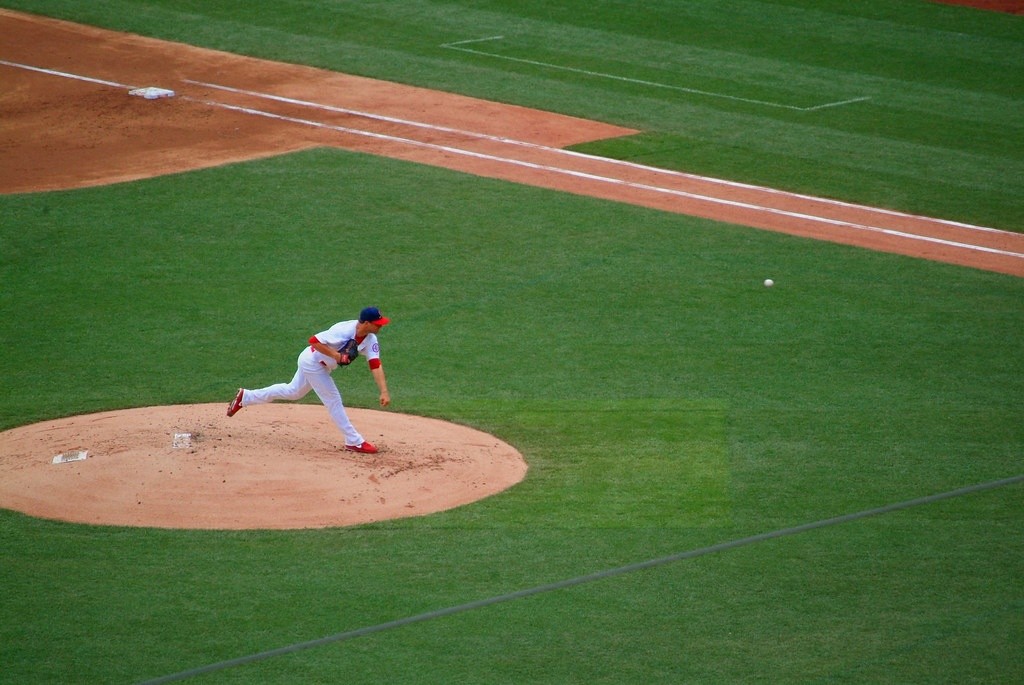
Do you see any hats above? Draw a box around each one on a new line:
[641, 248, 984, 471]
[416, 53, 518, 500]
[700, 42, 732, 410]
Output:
[360, 307, 390, 325]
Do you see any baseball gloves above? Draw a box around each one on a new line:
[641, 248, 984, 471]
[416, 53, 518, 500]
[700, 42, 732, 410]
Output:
[334, 338, 360, 368]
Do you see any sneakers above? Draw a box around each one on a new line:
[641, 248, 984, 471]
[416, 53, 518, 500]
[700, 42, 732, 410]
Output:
[226, 388, 244, 417]
[345, 441, 377, 453]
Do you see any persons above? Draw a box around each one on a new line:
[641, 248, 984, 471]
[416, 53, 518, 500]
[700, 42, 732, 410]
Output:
[227, 307, 389, 454]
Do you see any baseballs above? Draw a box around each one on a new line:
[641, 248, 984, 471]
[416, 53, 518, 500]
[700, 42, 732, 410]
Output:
[765, 278, 773, 287]
